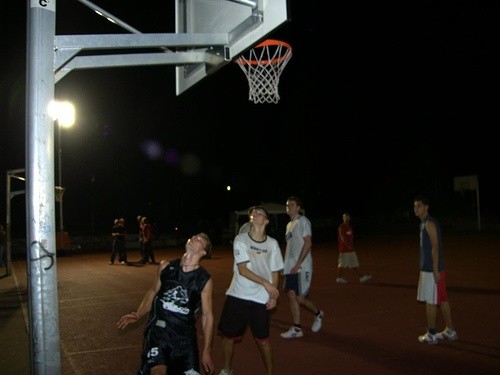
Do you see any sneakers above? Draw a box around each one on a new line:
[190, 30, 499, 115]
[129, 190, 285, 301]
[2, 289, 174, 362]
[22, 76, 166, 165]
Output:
[279, 324, 304, 338]
[311, 309, 323, 332]
[334, 276, 349, 283]
[437, 326, 459, 341]
[218, 367, 234, 375]
[418, 332, 438, 344]
[359, 272, 372, 282]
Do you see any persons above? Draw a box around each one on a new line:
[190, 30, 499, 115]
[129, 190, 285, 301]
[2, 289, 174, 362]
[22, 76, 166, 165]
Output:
[116, 232, 214, 375]
[217, 205, 284, 375]
[108, 216, 155, 265]
[280, 196, 326, 339]
[413, 197, 459, 345]
[335, 213, 374, 283]
[0, 223, 16, 278]
